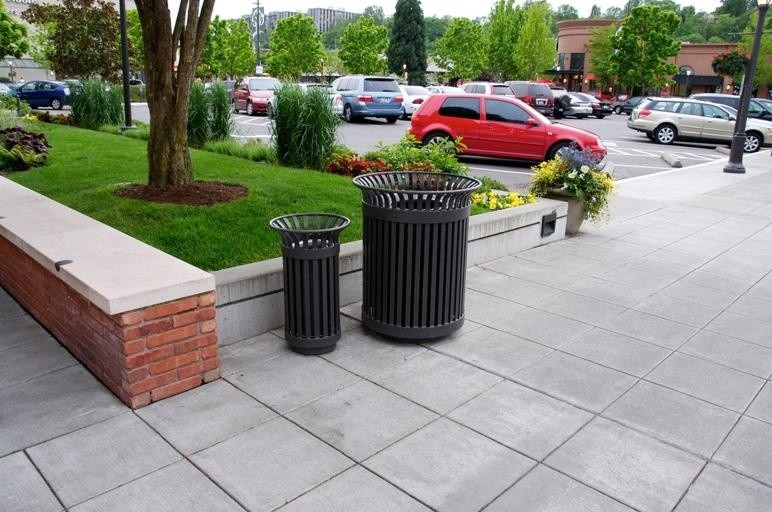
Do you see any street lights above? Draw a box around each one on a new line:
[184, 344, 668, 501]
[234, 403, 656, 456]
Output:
[319, 57, 325, 76]
[402, 63, 407, 80]
[685, 68, 692, 97]
[556, 66, 561, 82]
[6, 60, 14, 81]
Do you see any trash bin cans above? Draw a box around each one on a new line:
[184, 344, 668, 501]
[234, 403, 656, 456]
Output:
[270, 212, 352, 357]
[353, 171, 482, 345]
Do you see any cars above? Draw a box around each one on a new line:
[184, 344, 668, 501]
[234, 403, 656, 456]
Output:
[404, 90, 609, 170]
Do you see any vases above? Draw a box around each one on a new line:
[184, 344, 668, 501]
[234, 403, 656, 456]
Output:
[542, 185, 588, 237]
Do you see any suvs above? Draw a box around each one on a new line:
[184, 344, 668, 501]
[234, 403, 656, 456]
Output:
[623, 95, 772, 152]
[689, 92, 772, 123]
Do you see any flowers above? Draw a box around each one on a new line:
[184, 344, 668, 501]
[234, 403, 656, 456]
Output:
[520, 137, 619, 231]
